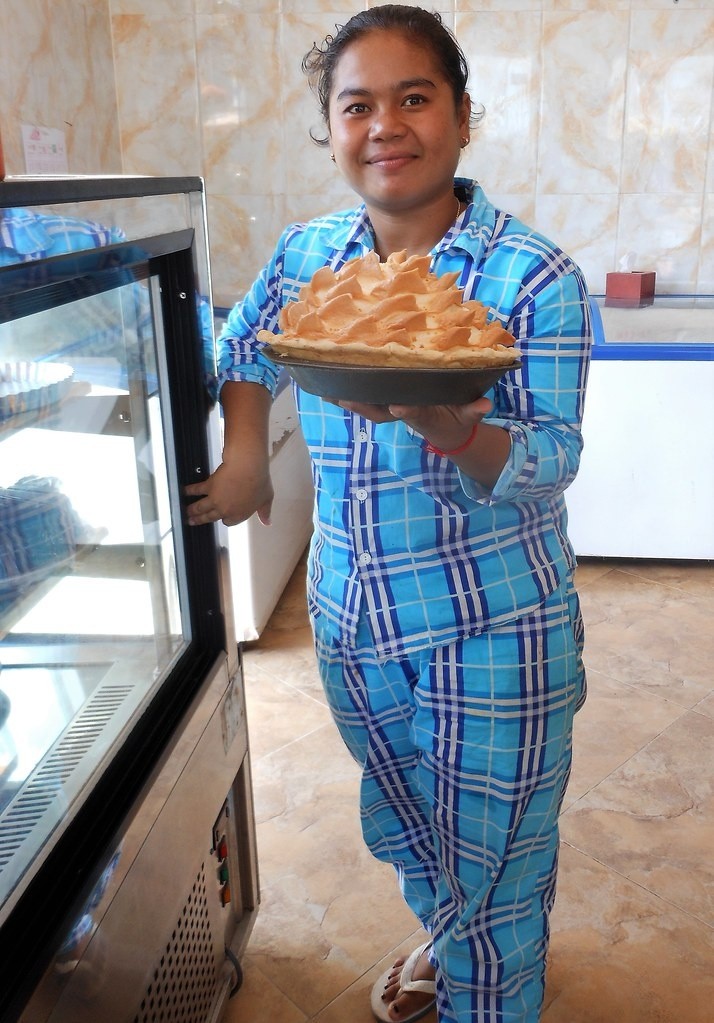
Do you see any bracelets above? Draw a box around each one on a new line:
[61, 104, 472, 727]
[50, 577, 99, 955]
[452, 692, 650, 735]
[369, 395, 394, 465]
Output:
[421, 425, 479, 457]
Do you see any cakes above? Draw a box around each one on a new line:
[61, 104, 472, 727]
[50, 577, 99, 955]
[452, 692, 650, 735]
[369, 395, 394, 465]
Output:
[255, 249, 525, 370]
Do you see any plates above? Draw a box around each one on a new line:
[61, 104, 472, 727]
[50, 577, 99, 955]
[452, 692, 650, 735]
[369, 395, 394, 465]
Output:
[0, 362, 72, 414]
[262, 345, 524, 406]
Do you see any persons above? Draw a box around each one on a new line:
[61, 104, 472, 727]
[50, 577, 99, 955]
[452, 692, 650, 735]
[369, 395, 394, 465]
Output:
[0, 205, 218, 1006]
[182, 5, 591, 1023]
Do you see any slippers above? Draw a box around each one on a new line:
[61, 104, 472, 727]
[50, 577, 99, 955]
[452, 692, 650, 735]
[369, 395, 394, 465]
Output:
[371, 940, 436, 1023]
